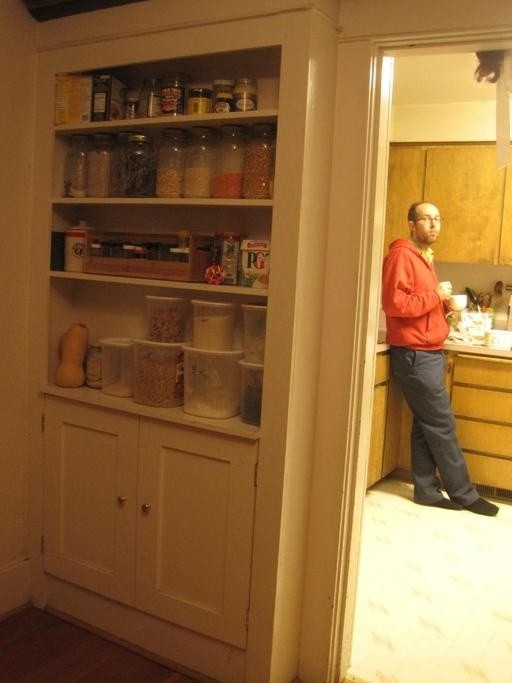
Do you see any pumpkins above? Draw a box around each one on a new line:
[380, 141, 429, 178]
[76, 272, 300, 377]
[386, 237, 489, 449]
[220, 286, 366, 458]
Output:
[56, 323, 89, 387]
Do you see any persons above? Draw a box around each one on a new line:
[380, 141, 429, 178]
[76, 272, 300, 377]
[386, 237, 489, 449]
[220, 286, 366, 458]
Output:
[381, 201, 500, 517]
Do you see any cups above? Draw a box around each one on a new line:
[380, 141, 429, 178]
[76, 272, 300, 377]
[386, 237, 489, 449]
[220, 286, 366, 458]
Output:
[448, 295, 494, 347]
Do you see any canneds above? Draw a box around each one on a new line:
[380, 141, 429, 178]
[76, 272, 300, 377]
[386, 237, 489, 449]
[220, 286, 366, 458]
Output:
[124, 97, 139, 119]
[160, 79, 185, 116]
[187, 77, 257, 115]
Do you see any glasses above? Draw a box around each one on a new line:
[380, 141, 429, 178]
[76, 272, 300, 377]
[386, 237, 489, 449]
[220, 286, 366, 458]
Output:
[411, 215, 443, 223]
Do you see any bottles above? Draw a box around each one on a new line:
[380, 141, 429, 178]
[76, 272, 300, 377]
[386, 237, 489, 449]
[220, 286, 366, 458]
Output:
[88, 229, 190, 264]
[98, 293, 268, 430]
[64, 219, 88, 274]
[124, 72, 258, 117]
[57, 132, 277, 200]
[213, 230, 245, 286]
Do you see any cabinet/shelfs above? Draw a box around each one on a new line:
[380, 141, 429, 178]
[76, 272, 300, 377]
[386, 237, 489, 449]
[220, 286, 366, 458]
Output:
[44, 41, 283, 299]
[384, 141, 507, 266]
[448, 353, 512, 507]
[499, 141, 512, 266]
[41, 386, 261, 682]
[367, 349, 404, 490]
[397, 351, 454, 474]
